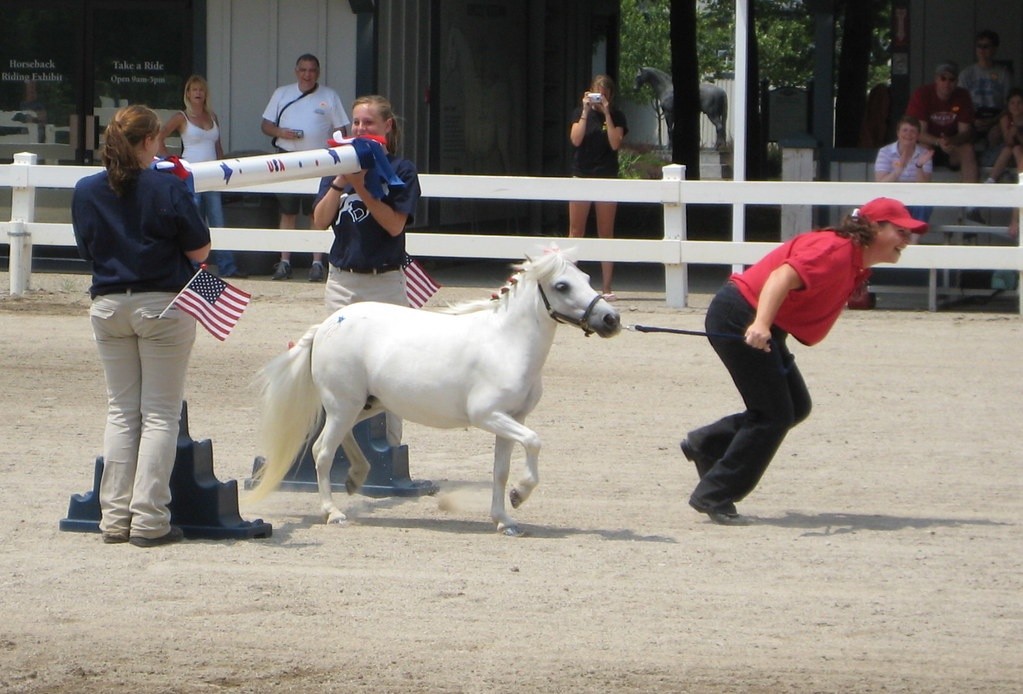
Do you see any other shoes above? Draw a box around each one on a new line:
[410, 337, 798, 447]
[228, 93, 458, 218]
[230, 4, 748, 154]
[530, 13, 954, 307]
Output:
[688, 500, 739, 526]
[130, 525, 183, 547]
[104, 534, 128, 543]
[681, 435, 711, 479]
[227, 269, 248, 278]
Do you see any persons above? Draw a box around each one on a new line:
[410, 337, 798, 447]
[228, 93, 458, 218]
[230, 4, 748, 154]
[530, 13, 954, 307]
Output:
[862, 84, 900, 146]
[570, 76, 629, 300]
[984, 87, 1023, 182]
[260, 53, 351, 280]
[312, 95, 422, 448]
[158, 75, 247, 277]
[958, 31, 1013, 166]
[875, 116, 934, 244]
[681, 197, 926, 526]
[1007, 208, 1019, 236]
[69, 105, 210, 549]
[908, 58, 985, 225]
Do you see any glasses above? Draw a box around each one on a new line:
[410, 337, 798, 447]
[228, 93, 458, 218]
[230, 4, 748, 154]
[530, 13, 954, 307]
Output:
[976, 42, 992, 49]
[940, 74, 957, 82]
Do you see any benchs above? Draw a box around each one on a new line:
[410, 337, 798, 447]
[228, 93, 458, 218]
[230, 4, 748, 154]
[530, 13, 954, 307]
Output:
[923, 225, 1019, 304]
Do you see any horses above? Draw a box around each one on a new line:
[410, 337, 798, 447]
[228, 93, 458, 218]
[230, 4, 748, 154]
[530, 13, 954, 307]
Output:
[231, 241, 623, 536]
[630, 66, 727, 151]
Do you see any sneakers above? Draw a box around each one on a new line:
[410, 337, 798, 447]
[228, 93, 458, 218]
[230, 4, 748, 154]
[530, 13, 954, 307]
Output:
[272, 261, 294, 281]
[308, 263, 325, 282]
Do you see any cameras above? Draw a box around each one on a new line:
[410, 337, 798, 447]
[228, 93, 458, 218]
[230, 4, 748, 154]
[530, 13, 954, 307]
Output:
[588, 93, 602, 104]
[292, 129, 303, 138]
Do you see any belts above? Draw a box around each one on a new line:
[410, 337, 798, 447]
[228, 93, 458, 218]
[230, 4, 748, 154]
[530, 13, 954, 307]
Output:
[342, 265, 401, 275]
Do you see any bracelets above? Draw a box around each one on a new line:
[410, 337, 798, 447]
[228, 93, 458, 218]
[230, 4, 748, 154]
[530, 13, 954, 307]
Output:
[580, 118, 587, 119]
[330, 183, 343, 191]
[915, 164, 922, 168]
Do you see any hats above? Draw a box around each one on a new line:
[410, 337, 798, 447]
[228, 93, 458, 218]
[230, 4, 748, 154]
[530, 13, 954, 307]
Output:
[856, 197, 927, 234]
[936, 63, 965, 74]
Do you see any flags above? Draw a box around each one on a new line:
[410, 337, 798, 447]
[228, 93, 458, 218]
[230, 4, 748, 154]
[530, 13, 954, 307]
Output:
[176, 269, 251, 341]
[404, 252, 440, 308]
